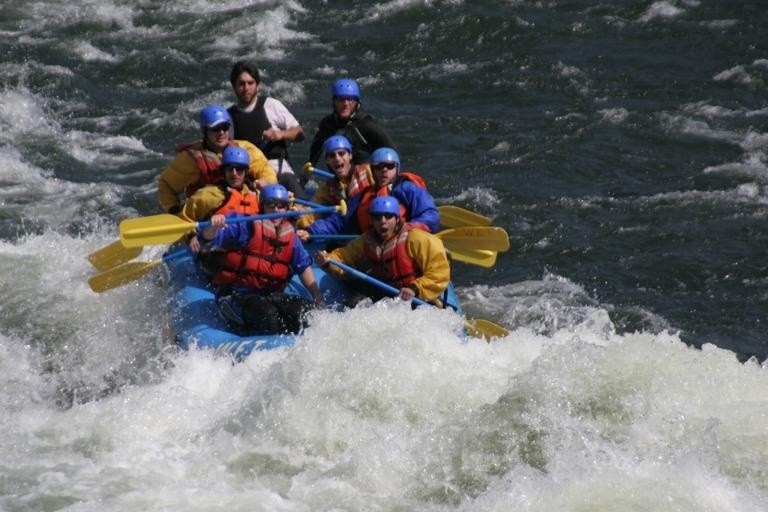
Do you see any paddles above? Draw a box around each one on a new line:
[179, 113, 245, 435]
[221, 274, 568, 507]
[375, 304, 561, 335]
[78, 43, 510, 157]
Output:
[86, 237, 212, 292]
[120, 200, 347, 248]
[329, 259, 508, 343]
[292, 163, 510, 268]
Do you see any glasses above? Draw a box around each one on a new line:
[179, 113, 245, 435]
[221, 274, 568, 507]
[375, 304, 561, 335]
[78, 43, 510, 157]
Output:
[335, 96, 356, 102]
[327, 151, 349, 159]
[207, 123, 231, 133]
[371, 162, 398, 170]
[264, 199, 288, 209]
[372, 212, 394, 221]
[222, 164, 245, 173]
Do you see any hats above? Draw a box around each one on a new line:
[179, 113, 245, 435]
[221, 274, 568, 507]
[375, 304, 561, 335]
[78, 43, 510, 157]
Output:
[367, 195, 401, 218]
[258, 183, 289, 205]
[321, 134, 353, 157]
[199, 103, 232, 129]
[369, 146, 401, 177]
[331, 78, 361, 101]
[221, 146, 250, 169]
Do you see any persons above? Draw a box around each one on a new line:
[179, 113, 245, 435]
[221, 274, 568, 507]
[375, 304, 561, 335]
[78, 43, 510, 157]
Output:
[197, 183, 323, 333]
[180, 150, 294, 289]
[310, 79, 396, 181]
[317, 195, 450, 310]
[174, 61, 307, 192]
[299, 162, 320, 200]
[296, 147, 438, 246]
[286, 136, 376, 228]
[161, 106, 279, 246]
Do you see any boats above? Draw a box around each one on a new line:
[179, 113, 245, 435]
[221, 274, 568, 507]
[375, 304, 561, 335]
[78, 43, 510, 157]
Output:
[160, 198, 468, 359]
